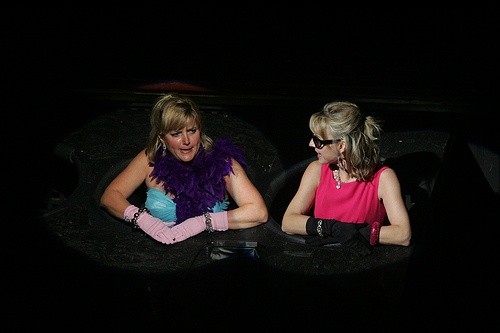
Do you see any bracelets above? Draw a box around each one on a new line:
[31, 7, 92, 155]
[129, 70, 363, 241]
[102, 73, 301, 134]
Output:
[370, 222, 382, 245]
[204, 212, 213, 233]
[317, 220, 324, 236]
[131, 207, 152, 229]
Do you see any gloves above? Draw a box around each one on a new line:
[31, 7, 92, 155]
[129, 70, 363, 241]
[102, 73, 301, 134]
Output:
[305, 224, 371, 247]
[306, 216, 373, 257]
[124, 204, 176, 245]
[170, 211, 228, 243]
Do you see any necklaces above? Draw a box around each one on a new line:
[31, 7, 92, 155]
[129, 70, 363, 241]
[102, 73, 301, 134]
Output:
[334, 170, 340, 189]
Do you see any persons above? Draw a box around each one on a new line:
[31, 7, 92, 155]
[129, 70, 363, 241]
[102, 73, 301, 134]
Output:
[281, 101, 412, 247]
[100, 94, 269, 245]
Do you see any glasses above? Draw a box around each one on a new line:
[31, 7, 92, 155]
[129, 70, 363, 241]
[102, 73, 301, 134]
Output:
[312, 134, 342, 148]
